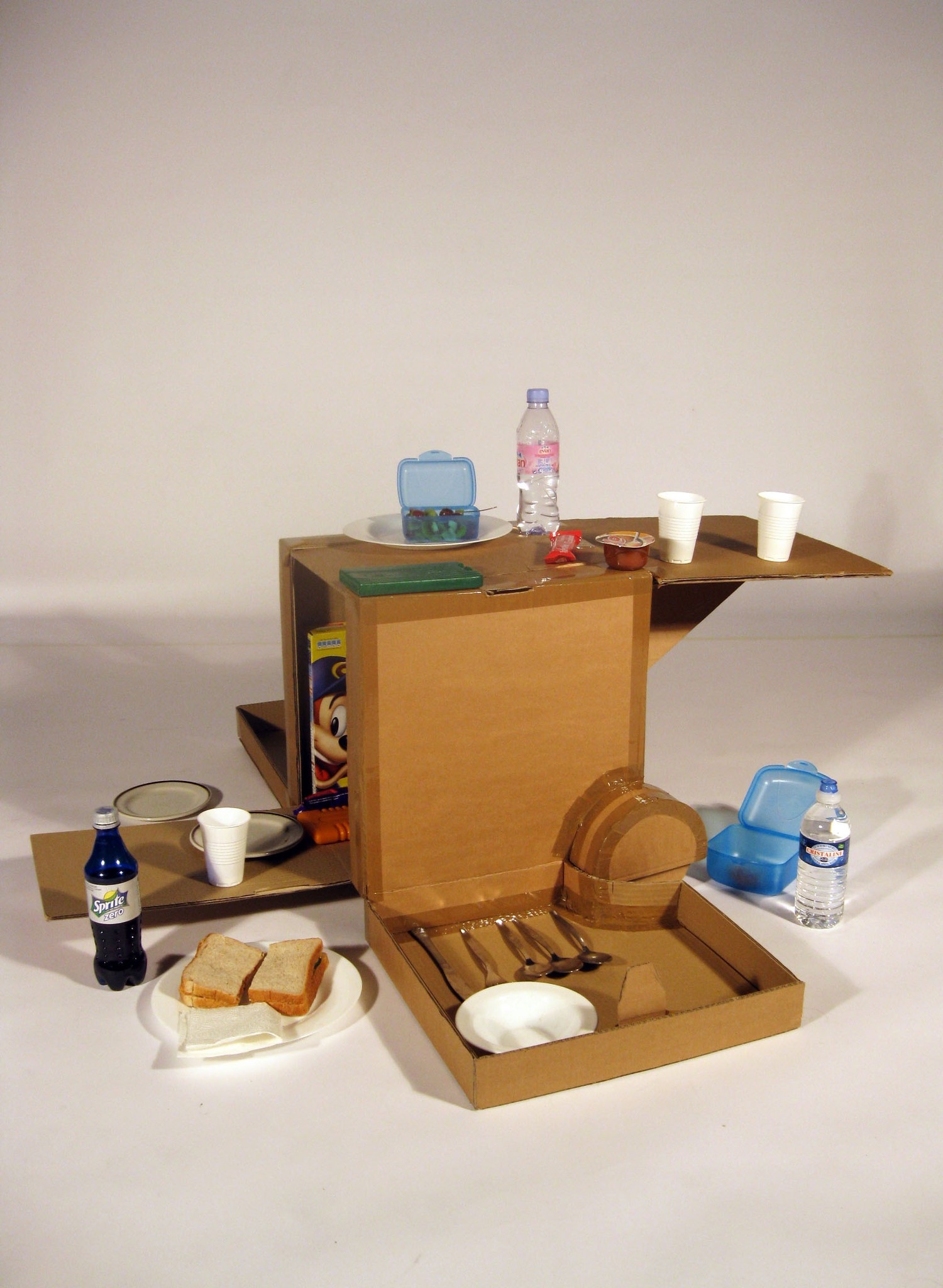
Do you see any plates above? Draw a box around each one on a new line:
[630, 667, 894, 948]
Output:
[113, 780, 213, 823]
[455, 981, 601, 1055]
[189, 809, 304, 859]
[344, 507, 514, 552]
[150, 940, 363, 1054]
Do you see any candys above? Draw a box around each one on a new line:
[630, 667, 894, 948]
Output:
[544, 530, 582, 563]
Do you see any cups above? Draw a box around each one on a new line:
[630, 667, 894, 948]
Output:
[755, 491, 806, 562]
[656, 490, 706, 564]
[197, 807, 251, 887]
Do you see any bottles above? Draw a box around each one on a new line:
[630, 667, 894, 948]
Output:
[794, 778, 851, 929]
[515, 386, 561, 536]
[85, 805, 147, 991]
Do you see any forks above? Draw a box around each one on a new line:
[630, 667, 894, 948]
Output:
[460, 927, 507, 988]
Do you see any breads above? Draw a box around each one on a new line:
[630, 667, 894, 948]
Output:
[179, 932, 329, 1016]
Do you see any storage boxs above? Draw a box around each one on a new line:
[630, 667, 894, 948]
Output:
[397, 449, 481, 542]
[29, 515, 895, 1110]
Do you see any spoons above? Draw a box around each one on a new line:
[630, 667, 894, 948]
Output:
[512, 918, 584, 972]
[552, 910, 613, 964]
[496, 918, 556, 976]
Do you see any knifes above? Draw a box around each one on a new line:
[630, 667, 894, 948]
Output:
[413, 926, 475, 1001]
